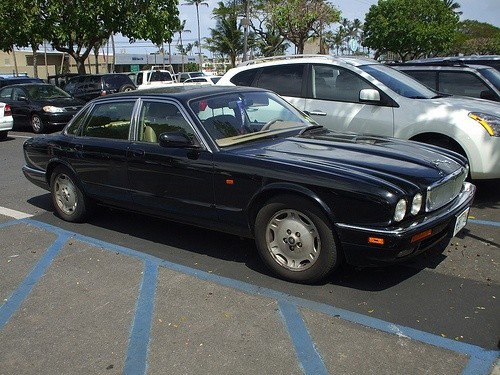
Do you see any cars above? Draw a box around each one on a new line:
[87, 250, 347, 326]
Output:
[1, 83, 87, 131]
[184, 76, 223, 85]
[1, 102, 14, 132]
[24, 86, 475, 284]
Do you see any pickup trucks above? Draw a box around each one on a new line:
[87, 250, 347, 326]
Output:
[134, 64, 210, 87]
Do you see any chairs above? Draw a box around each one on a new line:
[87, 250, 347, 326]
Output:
[143, 102, 181, 142]
[336, 70, 362, 101]
[205, 114, 245, 139]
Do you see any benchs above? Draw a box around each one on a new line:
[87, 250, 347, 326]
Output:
[83, 111, 157, 141]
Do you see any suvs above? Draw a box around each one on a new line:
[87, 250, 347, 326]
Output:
[65, 73, 136, 100]
[394, 64, 500, 102]
[216, 54, 500, 179]
[176, 71, 206, 82]
